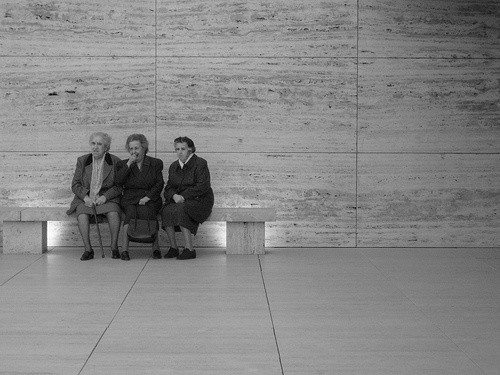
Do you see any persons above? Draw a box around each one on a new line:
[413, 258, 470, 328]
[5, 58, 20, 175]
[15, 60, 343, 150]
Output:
[161, 136, 214, 260]
[66, 132, 124, 261]
[113, 134, 165, 261]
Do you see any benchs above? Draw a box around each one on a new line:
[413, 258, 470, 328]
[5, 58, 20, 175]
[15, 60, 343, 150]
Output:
[0, 207, 277, 255]
